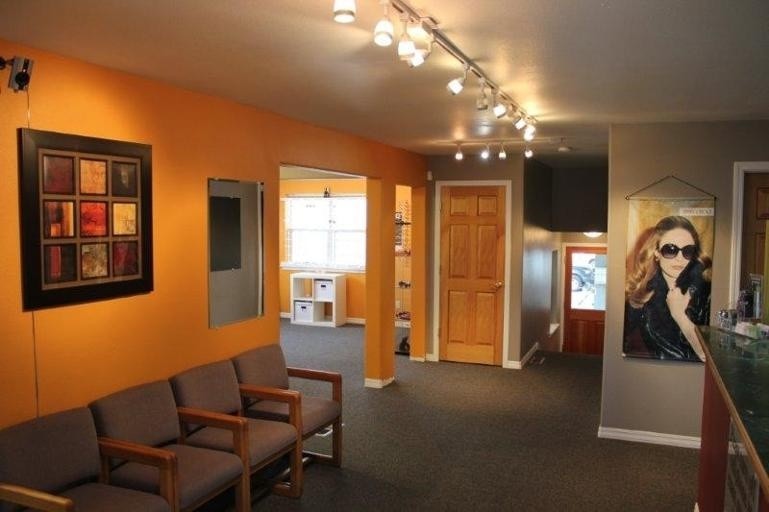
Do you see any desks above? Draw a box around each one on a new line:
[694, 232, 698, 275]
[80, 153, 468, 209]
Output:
[694, 318, 769, 512]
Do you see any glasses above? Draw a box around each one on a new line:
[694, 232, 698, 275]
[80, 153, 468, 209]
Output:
[660, 244, 698, 259]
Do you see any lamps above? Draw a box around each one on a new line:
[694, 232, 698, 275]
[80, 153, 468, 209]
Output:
[332, 0, 538, 142]
[453, 137, 549, 160]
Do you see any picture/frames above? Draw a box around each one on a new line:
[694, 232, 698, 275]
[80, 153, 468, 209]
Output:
[17, 127, 153, 311]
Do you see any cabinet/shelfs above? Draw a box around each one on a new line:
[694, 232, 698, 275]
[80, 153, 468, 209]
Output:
[290, 273, 347, 328]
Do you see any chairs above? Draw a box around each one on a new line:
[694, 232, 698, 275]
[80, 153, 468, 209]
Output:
[87, 376, 245, 511]
[167, 357, 300, 509]
[1, 405, 176, 511]
[235, 344, 339, 492]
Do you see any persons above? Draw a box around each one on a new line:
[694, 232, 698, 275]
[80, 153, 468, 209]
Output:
[624, 215, 713, 362]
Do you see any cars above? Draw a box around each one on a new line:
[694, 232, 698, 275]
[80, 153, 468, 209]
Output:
[571, 264, 594, 293]
